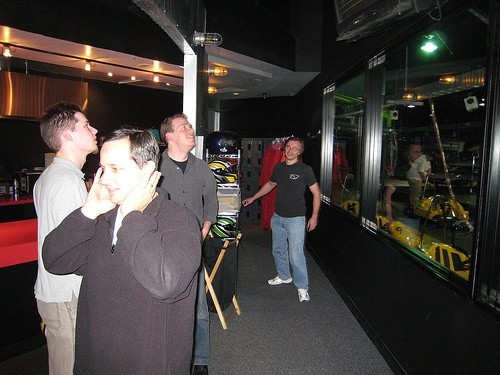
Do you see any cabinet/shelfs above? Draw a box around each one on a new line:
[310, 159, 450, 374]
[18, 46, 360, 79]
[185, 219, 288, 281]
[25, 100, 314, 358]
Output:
[242, 138, 271, 223]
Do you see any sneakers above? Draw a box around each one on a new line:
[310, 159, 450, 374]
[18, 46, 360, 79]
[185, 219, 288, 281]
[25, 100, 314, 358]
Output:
[267, 275, 292, 286]
[297, 288, 311, 303]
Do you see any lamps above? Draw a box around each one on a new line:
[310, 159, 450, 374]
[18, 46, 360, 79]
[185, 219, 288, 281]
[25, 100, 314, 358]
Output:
[208, 87, 218, 93]
[208, 66, 228, 77]
[194, 32, 222, 46]
[422, 32, 438, 52]
[3, 43, 12, 57]
[84, 59, 91, 72]
[153, 75, 159, 82]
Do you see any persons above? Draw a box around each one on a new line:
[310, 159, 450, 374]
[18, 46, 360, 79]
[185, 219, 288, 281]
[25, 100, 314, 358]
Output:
[242, 137, 321, 302]
[33, 101, 220, 375]
[384, 142, 439, 215]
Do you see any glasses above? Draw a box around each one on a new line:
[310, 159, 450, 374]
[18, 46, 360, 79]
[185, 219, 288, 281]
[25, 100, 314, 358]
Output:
[284, 146, 300, 152]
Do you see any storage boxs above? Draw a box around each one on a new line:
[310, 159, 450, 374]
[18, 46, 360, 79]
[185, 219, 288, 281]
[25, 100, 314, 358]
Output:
[217, 188, 241, 212]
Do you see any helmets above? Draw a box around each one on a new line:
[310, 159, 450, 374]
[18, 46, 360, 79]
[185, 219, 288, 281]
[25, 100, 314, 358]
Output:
[208, 158, 238, 183]
[206, 131, 239, 154]
[210, 217, 242, 241]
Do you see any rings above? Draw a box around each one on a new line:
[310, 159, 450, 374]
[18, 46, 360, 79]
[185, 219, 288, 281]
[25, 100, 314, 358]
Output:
[147, 182, 153, 187]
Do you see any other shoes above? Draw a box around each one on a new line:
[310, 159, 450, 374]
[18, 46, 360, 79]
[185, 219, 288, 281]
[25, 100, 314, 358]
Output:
[194, 365, 208, 375]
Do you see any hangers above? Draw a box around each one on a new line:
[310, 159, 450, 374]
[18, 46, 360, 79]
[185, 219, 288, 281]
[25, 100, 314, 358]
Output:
[273, 137, 291, 143]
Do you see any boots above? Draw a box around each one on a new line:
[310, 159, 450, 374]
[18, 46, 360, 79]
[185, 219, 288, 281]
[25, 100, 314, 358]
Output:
[404, 205, 418, 219]
